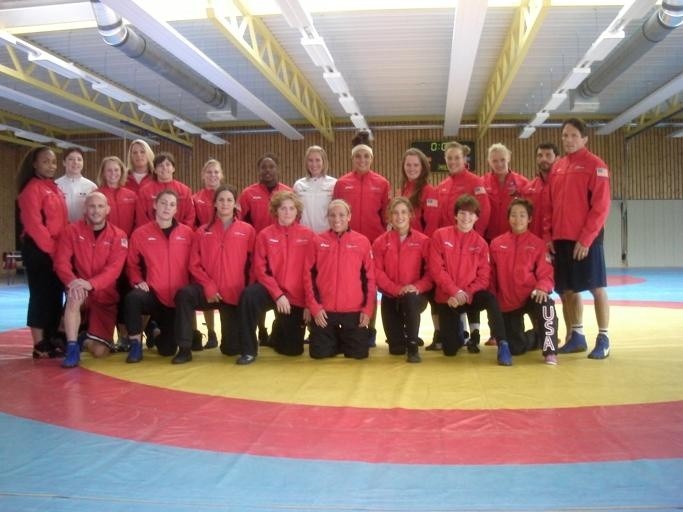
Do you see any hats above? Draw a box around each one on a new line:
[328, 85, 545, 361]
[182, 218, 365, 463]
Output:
[351, 130, 374, 159]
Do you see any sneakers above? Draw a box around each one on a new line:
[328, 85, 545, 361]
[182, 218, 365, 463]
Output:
[171, 340, 192, 364]
[467, 334, 479, 352]
[62, 344, 80, 368]
[485, 336, 497, 345]
[204, 329, 218, 348]
[544, 351, 557, 365]
[237, 353, 255, 364]
[258, 329, 269, 345]
[33, 343, 64, 359]
[588, 334, 610, 359]
[46, 336, 66, 345]
[425, 338, 445, 350]
[192, 329, 203, 351]
[497, 341, 512, 364]
[78, 331, 87, 348]
[145, 320, 157, 350]
[558, 330, 588, 353]
[127, 343, 143, 362]
[406, 344, 423, 362]
[118, 338, 127, 351]
[369, 327, 376, 346]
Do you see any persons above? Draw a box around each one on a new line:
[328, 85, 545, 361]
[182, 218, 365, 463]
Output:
[371, 196, 431, 362]
[434, 142, 492, 353]
[292, 145, 339, 235]
[125, 190, 195, 363]
[332, 131, 393, 349]
[138, 152, 203, 352]
[530, 143, 571, 346]
[16, 146, 69, 359]
[395, 148, 444, 351]
[236, 192, 315, 365]
[191, 158, 224, 349]
[489, 198, 559, 366]
[54, 147, 99, 223]
[53, 191, 129, 368]
[540, 117, 611, 359]
[235, 155, 292, 346]
[123, 139, 155, 194]
[480, 143, 530, 346]
[427, 193, 512, 366]
[171, 183, 257, 364]
[93, 157, 145, 352]
[303, 198, 377, 360]
